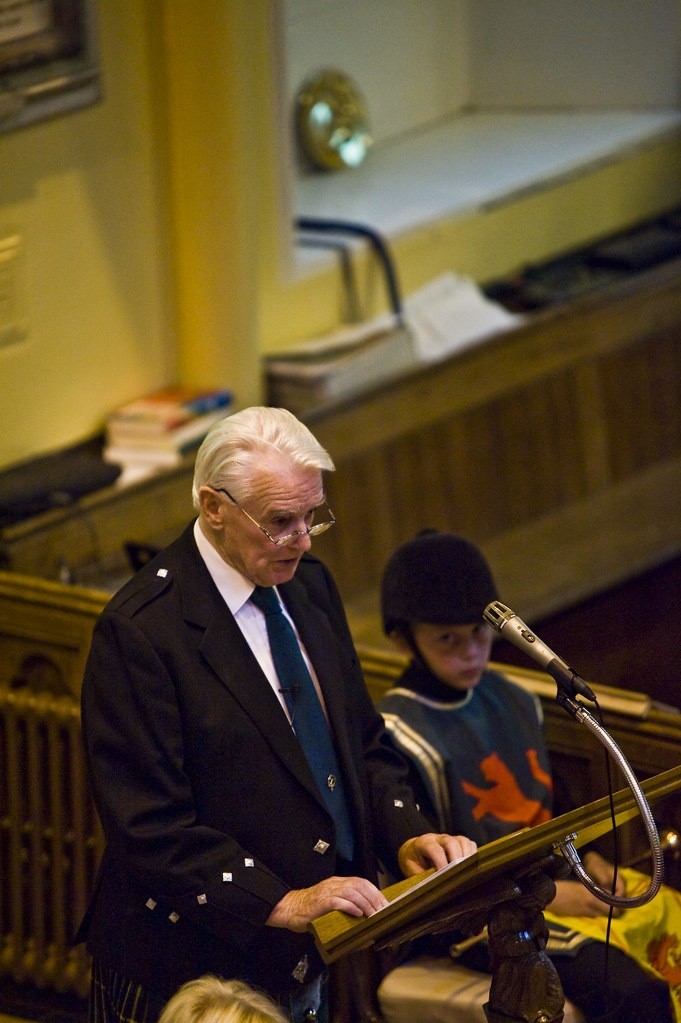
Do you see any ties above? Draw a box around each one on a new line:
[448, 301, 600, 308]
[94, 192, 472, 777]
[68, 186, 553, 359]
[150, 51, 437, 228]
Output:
[250, 585, 354, 863]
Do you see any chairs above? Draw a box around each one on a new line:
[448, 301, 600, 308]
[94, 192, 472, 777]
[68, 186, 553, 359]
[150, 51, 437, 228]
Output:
[335, 944, 589, 1023]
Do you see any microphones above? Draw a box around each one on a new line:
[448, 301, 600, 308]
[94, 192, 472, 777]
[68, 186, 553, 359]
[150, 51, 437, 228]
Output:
[483, 601, 596, 702]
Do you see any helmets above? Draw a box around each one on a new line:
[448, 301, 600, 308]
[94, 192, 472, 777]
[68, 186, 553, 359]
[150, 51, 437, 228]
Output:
[382, 529, 500, 631]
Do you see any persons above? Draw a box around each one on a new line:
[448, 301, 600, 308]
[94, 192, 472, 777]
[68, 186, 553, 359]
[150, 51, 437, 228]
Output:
[156, 974, 290, 1023]
[77, 406, 479, 1023]
[370, 525, 677, 1023]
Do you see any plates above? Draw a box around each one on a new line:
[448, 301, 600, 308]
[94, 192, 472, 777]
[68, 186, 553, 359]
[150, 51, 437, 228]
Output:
[295, 66, 371, 172]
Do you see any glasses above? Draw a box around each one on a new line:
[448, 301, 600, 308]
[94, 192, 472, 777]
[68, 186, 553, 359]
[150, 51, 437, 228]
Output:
[217, 489, 336, 545]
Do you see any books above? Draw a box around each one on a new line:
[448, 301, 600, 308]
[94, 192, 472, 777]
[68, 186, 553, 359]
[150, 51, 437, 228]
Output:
[268, 310, 412, 412]
[104, 381, 236, 469]
